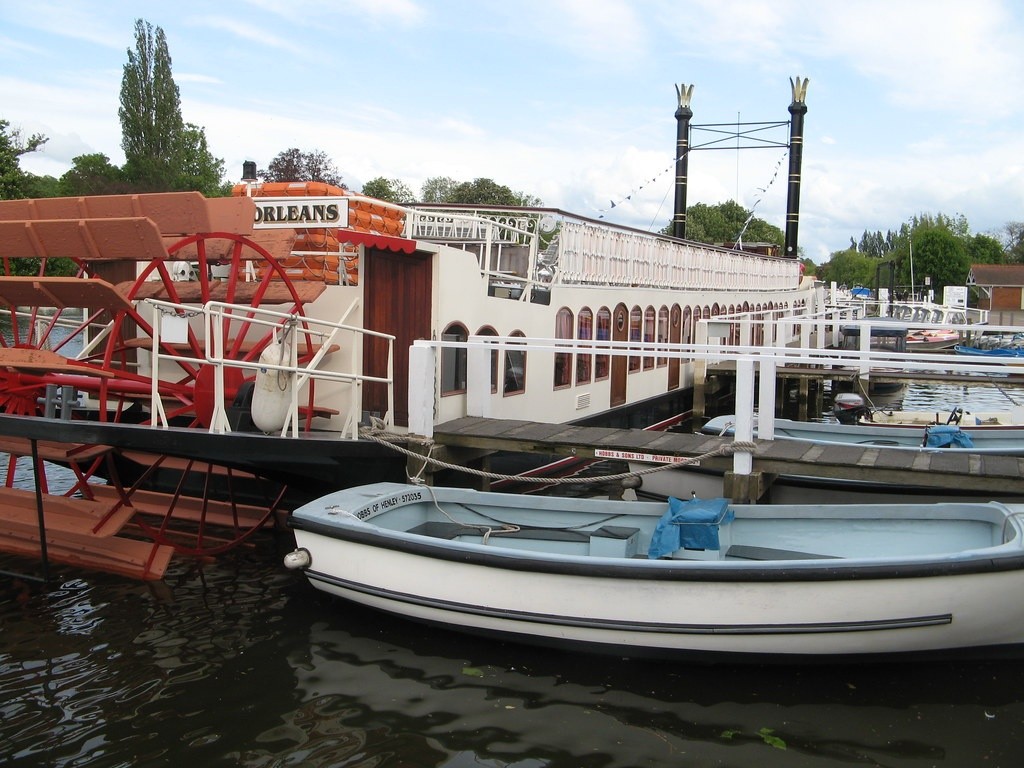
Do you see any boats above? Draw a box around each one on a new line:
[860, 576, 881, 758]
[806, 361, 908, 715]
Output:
[0, 76, 845, 600]
[627, 413, 1024, 503]
[283, 483, 1024, 654]
[954, 345, 1024, 358]
[833, 392, 1024, 430]
[871, 305, 984, 350]
